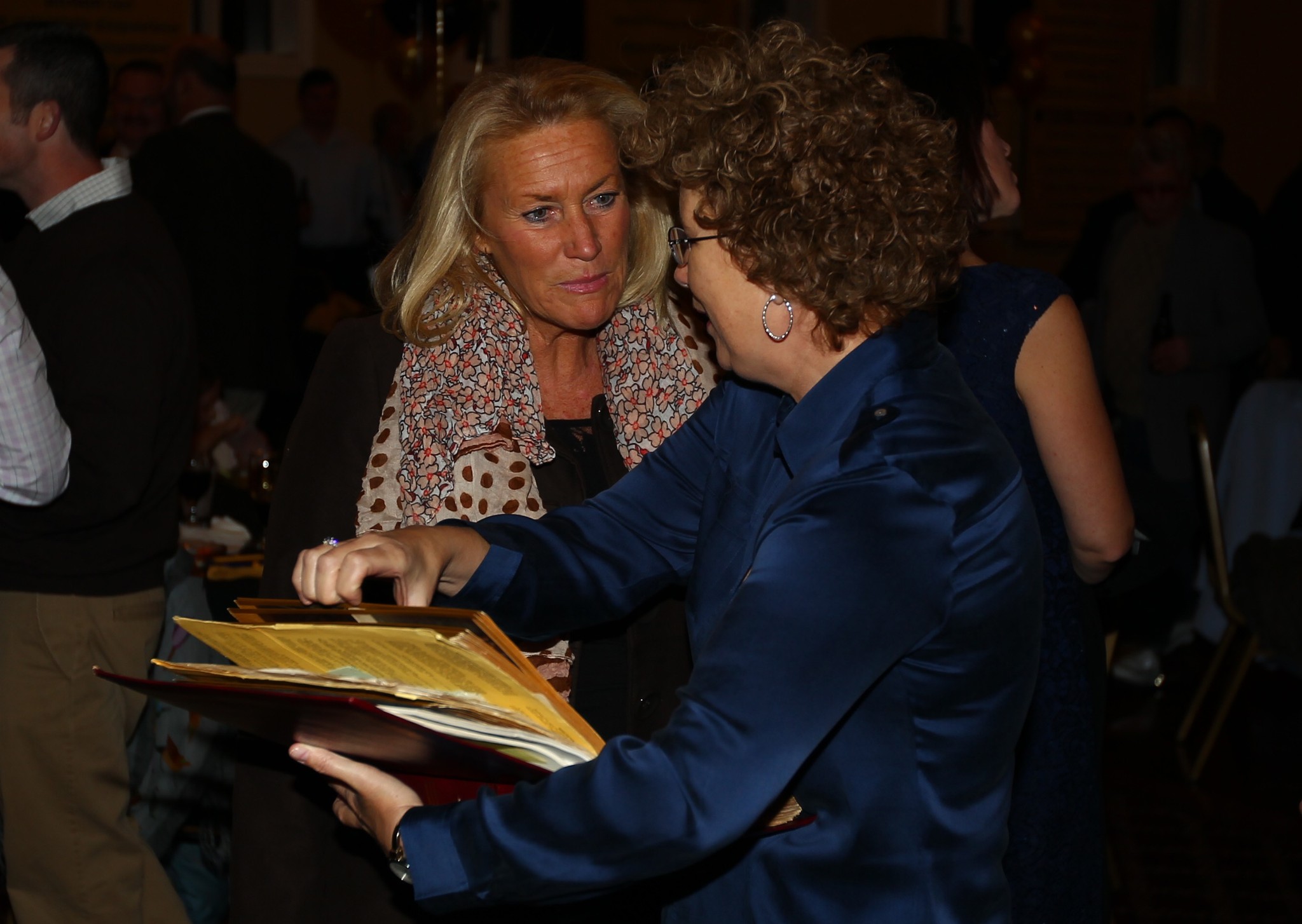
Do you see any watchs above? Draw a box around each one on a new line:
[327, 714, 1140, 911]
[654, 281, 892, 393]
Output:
[387, 818, 415, 886]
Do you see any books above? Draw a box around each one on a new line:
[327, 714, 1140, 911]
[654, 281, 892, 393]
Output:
[94, 598, 817, 838]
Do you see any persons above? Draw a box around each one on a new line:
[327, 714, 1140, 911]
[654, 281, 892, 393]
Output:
[0, 18, 204, 918]
[357, 56, 727, 752]
[104, 43, 442, 924]
[1050, 106, 1301, 691]
[0, 264, 72, 508]
[291, 17, 1047, 924]
[843, 33, 1132, 923]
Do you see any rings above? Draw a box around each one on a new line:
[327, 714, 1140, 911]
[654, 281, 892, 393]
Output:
[322, 537, 340, 549]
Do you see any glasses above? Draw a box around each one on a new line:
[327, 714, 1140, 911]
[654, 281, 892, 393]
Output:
[667, 225, 730, 267]
